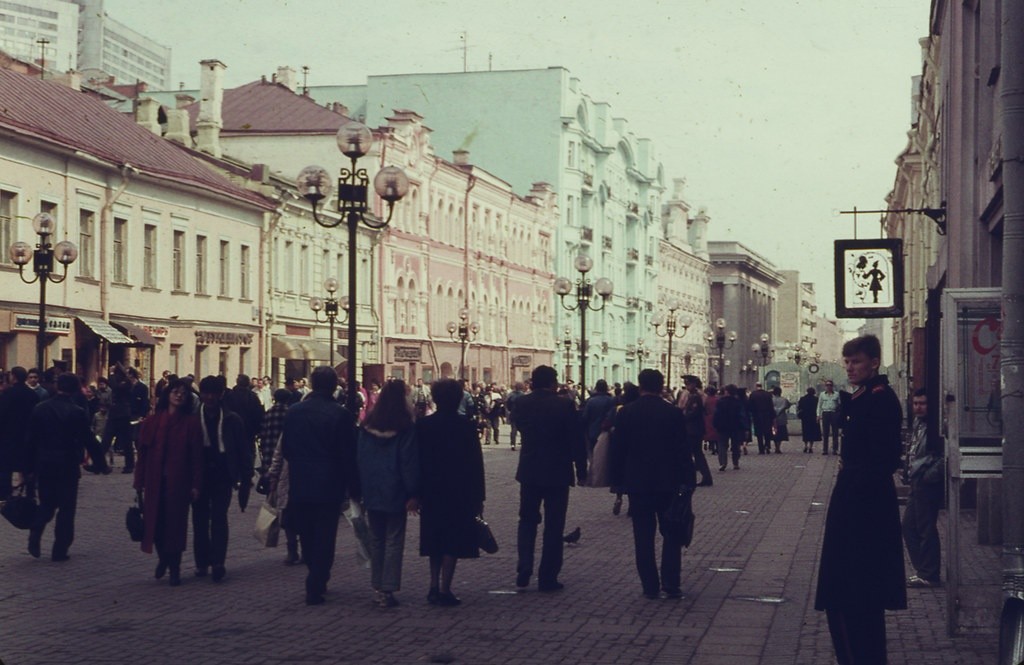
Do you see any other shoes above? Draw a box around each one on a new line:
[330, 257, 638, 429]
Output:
[379, 596, 399, 608]
[284, 553, 299, 564]
[169, 569, 181, 586]
[427, 589, 440, 601]
[440, 594, 461, 607]
[516, 567, 533, 587]
[908, 575, 940, 588]
[307, 589, 322, 606]
[212, 564, 225, 581]
[539, 579, 565, 591]
[154, 561, 167, 579]
[194, 563, 209, 577]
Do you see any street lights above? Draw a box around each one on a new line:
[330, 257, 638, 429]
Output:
[308, 277, 351, 366]
[786, 342, 808, 399]
[553, 254, 615, 407]
[649, 299, 691, 393]
[741, 359, 757, 391]
[447, 307, 480, 389]
[629, 337, 650, 372]
[704, 317, 738, 392]
[9, 211, 78, 382]
[751, 333, 777, 391]
[295, 120, 411, 422]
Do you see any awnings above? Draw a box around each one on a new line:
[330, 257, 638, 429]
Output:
[108, 320, 163, 348]
[271, 335, 336, 362]
[75, 315, 133, 347]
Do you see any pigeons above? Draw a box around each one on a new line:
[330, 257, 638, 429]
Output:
[170, 315, 179, 320]
[562, 525, 582, 548]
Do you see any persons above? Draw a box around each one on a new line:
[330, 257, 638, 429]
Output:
[796, 388, 822, 453]
[5, 362, 752, 515]
[606, 368, 696, 601]
[350, 378, 416, 607]
[510, 365, 588, 590]
[814, 332, 909, 665]
[19, 373, 112, 563]
[748, 382, 797, 454]
[816, 380, 841, 455]
[411, 378, 487, 604]
[280, 367, 357, 606]
[189, 376, 253, 579]
[901, 387, 942, 588]
[129, 379, 202, 584]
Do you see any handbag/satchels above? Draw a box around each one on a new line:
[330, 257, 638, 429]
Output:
[474, 513, 499, 554]
[2, 479, 51, 528]
[658, 492, 695, 549]
[126, 490, 145, 543]
[253, 490, 282, 549]
